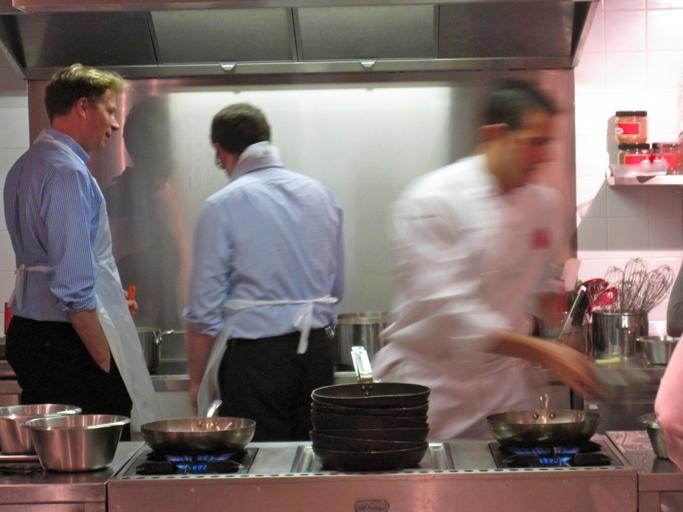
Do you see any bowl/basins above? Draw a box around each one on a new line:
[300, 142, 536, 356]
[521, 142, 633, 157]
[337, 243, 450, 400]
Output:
[637, 412, 670, 459]
[1, 403, 81, 456]
[637, 334, 679, 365]
[21, 414, 130, 472]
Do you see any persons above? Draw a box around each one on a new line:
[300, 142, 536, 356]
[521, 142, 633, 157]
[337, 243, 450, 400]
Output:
[183, 93, 346, 443]
[654, 258, 682, 471]
[1, 55, 142, 442]
[367, 77, 613, 443]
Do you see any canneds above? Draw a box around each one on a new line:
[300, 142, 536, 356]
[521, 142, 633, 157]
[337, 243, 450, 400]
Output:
[614, 110, 649, 145]
[650, 143, 677, 175]
[617, 144, 627, 164]
[623, 142, 650, 167]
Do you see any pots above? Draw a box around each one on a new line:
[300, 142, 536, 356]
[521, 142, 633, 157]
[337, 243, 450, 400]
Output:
[310, 346, 430, 470]
[486, 393, 599, 447]
[140, 399, 256, 456]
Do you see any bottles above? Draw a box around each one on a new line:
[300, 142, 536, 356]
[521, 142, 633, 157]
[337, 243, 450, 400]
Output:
[614, 142, 666, 174]
